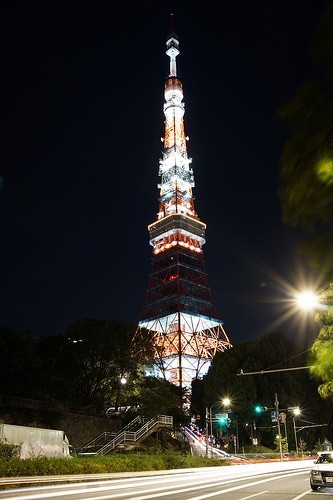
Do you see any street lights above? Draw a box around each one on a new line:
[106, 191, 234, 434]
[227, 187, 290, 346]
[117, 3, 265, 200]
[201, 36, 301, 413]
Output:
[292, 408, 301, 457]
[256, 407, 283, 460]
[206, 419, 224, 460]
[209, 399, 229, 458]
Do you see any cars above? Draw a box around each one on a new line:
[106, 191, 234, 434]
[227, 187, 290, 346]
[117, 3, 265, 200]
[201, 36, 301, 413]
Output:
[310, 451, 333, 491]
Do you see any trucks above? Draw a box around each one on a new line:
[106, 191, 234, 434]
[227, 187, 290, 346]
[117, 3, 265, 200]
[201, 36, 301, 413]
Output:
[0, 424, 78, 459]
